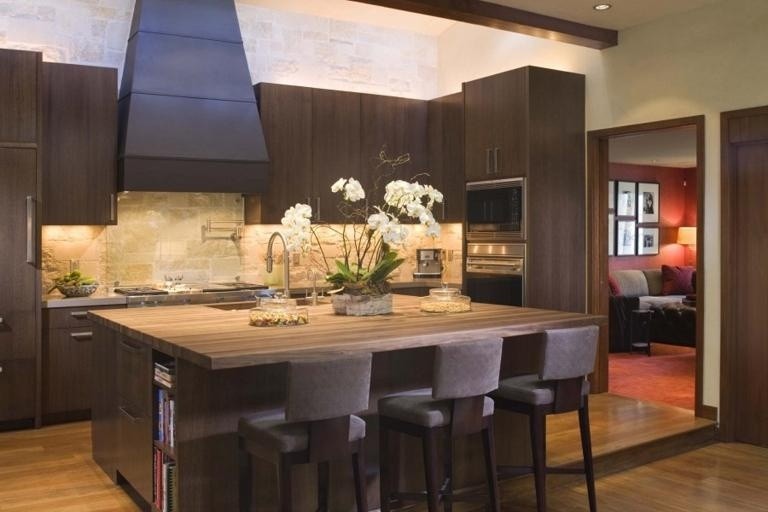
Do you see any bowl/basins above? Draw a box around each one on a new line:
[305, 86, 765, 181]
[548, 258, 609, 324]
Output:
[56, 283, 100, 298]
[428, 287, 461, 300]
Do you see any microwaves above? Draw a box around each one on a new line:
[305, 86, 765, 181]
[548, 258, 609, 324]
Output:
[463, 176, 526, 241]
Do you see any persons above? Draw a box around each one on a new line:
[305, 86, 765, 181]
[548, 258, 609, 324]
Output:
[644, 193, 654, 214]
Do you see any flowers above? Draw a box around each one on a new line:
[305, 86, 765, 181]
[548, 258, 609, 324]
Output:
[280, 148, 446, 290]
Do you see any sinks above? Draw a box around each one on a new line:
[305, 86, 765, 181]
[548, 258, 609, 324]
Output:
[205, 300, 330, 313]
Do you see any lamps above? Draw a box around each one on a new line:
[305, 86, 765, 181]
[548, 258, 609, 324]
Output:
[676, 227, 697, 266]
[680, 178, 688, 192]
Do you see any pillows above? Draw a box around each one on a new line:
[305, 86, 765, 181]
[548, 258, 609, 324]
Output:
[608, 270, 621, 296]
[660, 265, 693, 295]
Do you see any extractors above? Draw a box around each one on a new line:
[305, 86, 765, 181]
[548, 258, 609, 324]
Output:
[114, 0, 272, 194]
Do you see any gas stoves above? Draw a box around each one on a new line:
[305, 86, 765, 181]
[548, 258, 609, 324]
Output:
[113, 286, 168, 297]
[176, 280, 268, 292]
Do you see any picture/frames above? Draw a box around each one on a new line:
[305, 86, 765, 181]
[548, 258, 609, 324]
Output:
[636, 225, 660, 256]
[608, 213, 616, 258]
[616, 181, 636, 216]
[608, 179, 615, 210]
[616, 221, 637, 256]
[637, 180, 660, 224]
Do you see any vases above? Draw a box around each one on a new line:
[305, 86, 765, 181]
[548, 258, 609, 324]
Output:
[327, 290, 393, 317]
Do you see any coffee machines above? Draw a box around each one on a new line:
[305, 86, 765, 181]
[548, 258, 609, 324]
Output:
[412, 247, 443, 279]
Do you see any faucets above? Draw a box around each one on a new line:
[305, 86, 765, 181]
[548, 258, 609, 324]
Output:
[264, 232, 292, 299]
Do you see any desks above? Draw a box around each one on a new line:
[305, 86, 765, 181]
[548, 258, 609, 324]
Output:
[631, 309, 654, 357]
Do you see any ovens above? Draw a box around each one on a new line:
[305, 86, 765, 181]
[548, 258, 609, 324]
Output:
[464, 255, 525, 309]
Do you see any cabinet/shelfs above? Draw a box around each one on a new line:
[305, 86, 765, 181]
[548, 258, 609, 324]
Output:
[0, 48, 39, 432]
[360, 89, 427, 226]
[463, 64, 585, 181]
[40, 62, 118, 227]
[426, 89, 463, 224]
[114, 329, 156, 507]
[43, 305, 126, 414]
[244, 82, 359, 227]
[152, 348, 239, 511]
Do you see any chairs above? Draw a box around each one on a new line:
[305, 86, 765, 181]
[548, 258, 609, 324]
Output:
[487, 323, 602, 511]
[377, 335, 504, 512]
[238, 351, 372, 511]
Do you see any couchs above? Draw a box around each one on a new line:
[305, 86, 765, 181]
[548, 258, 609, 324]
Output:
[608, 264, 697, 352]
